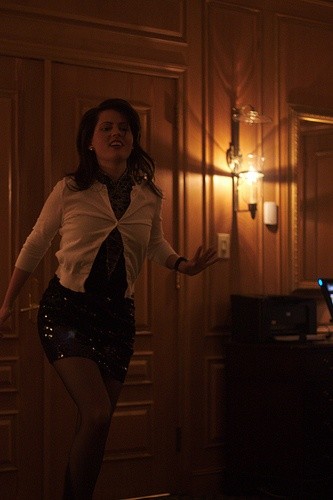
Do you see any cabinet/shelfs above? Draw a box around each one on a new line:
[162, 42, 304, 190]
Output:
[227, 332, 333, 500]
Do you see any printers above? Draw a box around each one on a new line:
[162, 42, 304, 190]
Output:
[229, 292, 316, 345]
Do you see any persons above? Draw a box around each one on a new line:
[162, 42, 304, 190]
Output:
[0, 98, 217, 500]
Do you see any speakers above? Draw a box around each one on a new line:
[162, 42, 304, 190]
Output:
[263, 201, 279, 226]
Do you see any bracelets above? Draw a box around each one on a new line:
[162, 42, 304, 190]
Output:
[174, 256, 189, 272]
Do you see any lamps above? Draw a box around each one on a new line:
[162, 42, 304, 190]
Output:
[233, 161, 265, 220]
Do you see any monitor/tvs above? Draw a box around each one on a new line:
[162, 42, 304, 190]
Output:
[318, 278, 333, 322]
